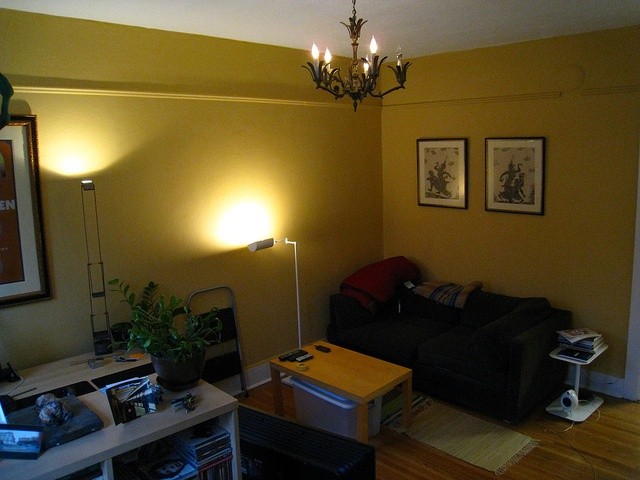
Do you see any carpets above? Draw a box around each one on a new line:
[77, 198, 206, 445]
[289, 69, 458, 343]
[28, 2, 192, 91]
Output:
[380, 384, 543, 476]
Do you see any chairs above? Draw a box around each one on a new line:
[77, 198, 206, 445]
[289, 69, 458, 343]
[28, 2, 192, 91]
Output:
[186, 284, 250, 399]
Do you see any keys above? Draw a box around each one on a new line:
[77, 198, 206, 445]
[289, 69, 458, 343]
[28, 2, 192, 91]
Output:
[170, 391, 198, 415]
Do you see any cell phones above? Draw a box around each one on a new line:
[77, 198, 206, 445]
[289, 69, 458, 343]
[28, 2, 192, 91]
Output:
[314, 344, 331, 353]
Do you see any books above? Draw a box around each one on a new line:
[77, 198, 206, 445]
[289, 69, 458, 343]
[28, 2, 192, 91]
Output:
[556, 326, 604, 353]
[172, 421, 233, 480]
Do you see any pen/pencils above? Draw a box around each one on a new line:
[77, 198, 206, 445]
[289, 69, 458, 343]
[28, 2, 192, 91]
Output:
[171, 393, 197, 413]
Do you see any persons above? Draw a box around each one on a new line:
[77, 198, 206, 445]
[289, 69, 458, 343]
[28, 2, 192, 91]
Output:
[146, 457, 186, 479]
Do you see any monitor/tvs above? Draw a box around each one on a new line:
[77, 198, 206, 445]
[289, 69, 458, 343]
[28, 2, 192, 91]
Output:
[237, 402, 376, 479]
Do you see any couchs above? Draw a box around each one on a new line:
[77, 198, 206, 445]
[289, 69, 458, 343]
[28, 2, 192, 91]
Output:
[328, 289, 571, 424]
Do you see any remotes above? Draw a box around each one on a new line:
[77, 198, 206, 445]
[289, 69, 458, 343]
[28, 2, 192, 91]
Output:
[288, 350, 308, 362]
[296, 354, 312, 361]
[277, 349, 302, 362]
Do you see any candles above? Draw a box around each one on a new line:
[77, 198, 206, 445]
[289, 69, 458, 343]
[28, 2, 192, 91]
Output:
[395, 41, 403, 69]
[369, 34, 378, 63]
[363, 54, 369, 75]
[323, 47, 333, 70]
[311, 42, 319, 71]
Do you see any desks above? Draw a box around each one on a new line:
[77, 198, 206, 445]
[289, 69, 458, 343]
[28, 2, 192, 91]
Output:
[0, 342, 243, 479]
[545, 343, 609, 423]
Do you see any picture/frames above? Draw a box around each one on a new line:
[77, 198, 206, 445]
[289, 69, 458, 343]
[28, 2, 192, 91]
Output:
[484, 136, 547, 216]
[1, 112, 54, 310]
[416, 136, 469, 210]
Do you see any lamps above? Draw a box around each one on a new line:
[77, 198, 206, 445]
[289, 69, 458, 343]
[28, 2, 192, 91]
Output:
[80, 179, 114, 355]
[301, 0, 412, 113]
[247, 237, 303, 349]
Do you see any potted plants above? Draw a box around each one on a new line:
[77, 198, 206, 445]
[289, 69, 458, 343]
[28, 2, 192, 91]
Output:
[106, 277, 223, 393]
